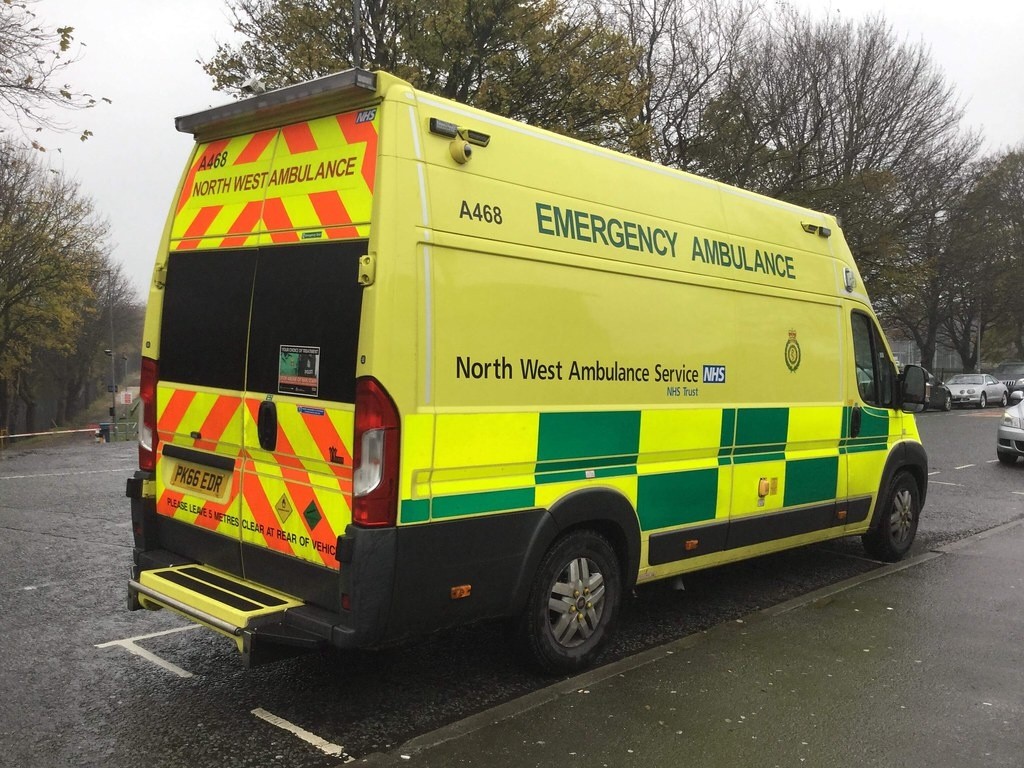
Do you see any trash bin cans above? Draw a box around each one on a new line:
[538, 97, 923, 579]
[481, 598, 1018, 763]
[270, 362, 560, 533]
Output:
[100, 423, 110, 442]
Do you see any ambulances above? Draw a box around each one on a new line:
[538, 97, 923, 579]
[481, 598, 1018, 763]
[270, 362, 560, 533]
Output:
[124, 66, 932, 675]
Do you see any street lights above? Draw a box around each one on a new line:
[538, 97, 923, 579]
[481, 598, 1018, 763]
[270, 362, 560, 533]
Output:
[101, 268, 117, 426]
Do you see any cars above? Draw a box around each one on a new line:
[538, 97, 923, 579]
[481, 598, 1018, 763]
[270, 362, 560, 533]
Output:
[928, 373, 953, 411]
[945, 373, 1009, 409]
[996, 390, 1024, 464]
